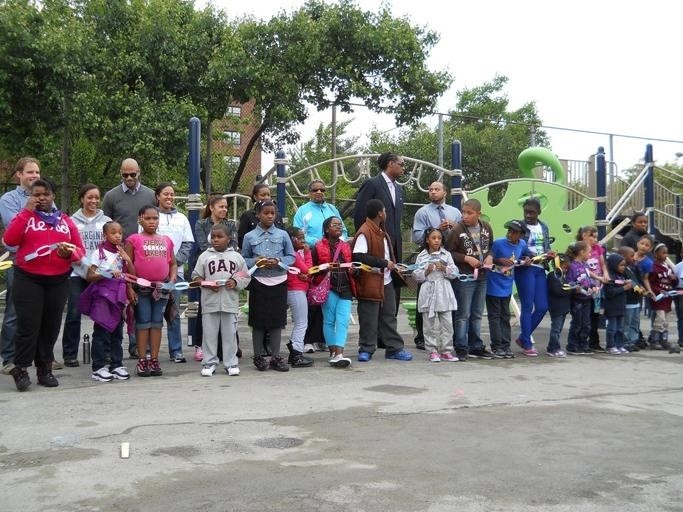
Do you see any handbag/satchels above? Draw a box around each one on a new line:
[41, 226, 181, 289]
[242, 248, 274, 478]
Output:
[306, 272, 333, 306]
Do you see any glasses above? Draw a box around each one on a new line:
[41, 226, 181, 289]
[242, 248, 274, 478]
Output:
[309, 188, 327, 193]
[122, 173, 137, 178]
[394, 162, 404, 167]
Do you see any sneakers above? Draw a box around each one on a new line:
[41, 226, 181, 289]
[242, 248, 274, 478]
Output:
[358, 351, 372, 361]
[11, 368, 32, 391]
[110, 367, 130, 381]
[146, 358, 163, 376]
[505, 220, 527, 233]
[37, 366, 59, 386]
[1, 358, 82, 373]
[136, 359, 150, 376]
[385, 348, 413, 361]
[201, 362, 217, 377]
[92, 367, 113, 382]
[254, 356, 268, 371]
[270, 356, 290, 371]
[417, 338, 682, 362]
[225, 365, 240, 375]
[287, 338, 352, 366]
[129, 336, 203, 362]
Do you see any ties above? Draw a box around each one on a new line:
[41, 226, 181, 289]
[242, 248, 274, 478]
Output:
[438, 206, 449, 235]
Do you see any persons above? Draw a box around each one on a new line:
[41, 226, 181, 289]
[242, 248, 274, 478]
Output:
[1, 179, 251, 393]
[353, 151, 408, 348]
[99, 157, 160, 359]
[413, 181, 683, 362]
[351, 199, 411, 361]
[239, 180, 363, 371]
[0, 158, 60, 377]
